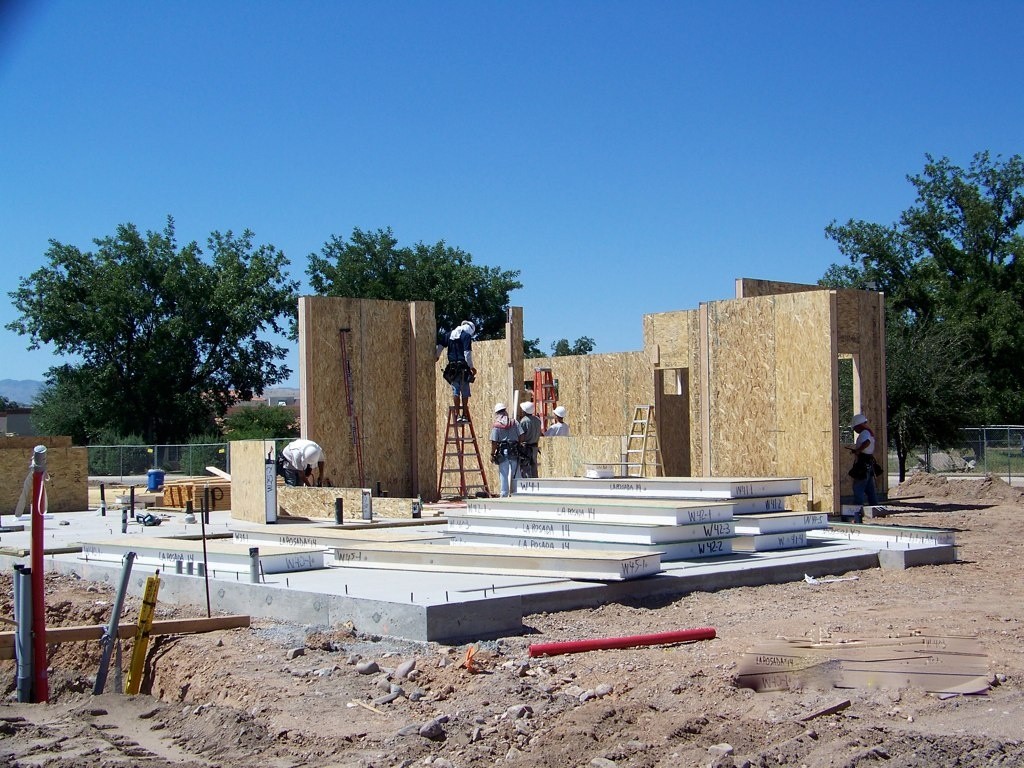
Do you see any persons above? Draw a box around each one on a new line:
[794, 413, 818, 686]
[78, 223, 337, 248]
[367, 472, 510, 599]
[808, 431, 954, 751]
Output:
[541, 406, 570, 436]
[278, 438, 326, 488]
[435, 320, 478, 425]
[844, 414, 883, 506]
[490, 402, 524, 499]
[516, 401, 542, 478]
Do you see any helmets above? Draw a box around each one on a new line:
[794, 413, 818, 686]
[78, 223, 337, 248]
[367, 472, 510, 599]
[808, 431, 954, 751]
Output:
[461, 320, 475, 332]
[302, 445, 321, 465]
[851, 414, 868, 428]
[552, 406, 567, 419]
[519, 400, 535, 415]
[494, 403, 506, 413]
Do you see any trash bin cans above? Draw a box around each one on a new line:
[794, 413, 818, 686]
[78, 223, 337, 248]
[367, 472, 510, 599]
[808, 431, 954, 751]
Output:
[146, 467, 166, 493]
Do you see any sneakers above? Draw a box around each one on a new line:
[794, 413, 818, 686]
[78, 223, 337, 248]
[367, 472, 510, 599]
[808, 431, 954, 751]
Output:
[456, 415, 462, 421]
[461, 415, 468, 421]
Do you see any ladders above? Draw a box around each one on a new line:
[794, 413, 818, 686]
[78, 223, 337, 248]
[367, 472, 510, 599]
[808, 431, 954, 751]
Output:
[436, 404, 493, 497]
[533, 366, 558, 437]
[622, 404, 668, 477]
